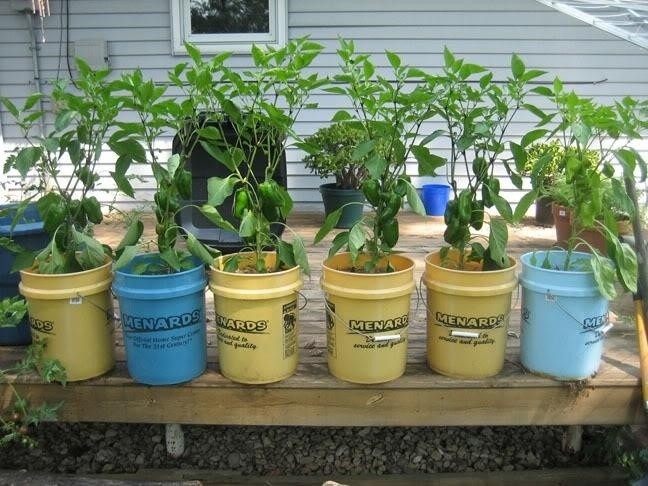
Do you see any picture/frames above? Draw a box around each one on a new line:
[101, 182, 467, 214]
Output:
[169, 0, 288, 56]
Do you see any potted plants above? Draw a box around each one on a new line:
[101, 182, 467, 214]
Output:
[517, 68, 636, 384]
[110, 52, 213, 385]
[2, 66, 102, 353]
[545, 143, 648, 254]
[297, 110, 367, 228]
[383, 39, 520, 379]
[297, 29, 416, 385]
[3, 53, 118, 382]
[173, 36, 311, 385]
[525, 135, 602, 230]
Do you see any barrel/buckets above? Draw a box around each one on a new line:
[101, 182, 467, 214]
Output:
[320, 250, 420, 385]
[208, 251, 307, 385]
[0, 204, 54, 347]
[520, 249, 616, 384]
[419, 247, 519, 381]
[112, 252, 209, 384]
[17, 250, 121, 383]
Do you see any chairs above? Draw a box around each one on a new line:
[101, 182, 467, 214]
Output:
[172, 110, 288, 263]
